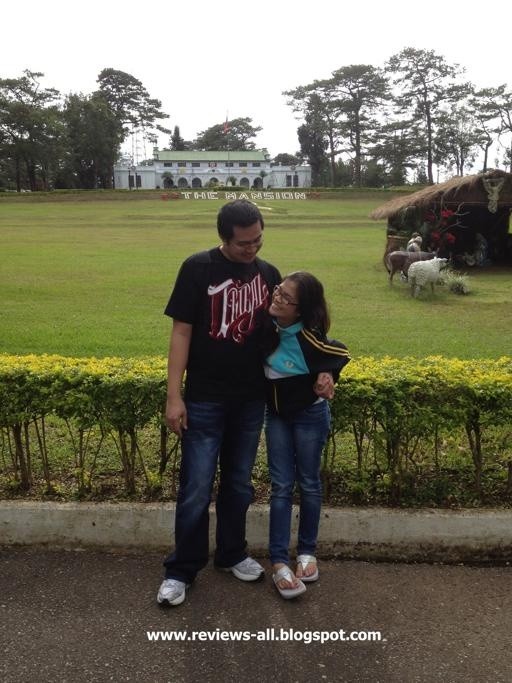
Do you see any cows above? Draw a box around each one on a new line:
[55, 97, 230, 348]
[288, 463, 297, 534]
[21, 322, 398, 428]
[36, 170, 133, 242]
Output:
[384, 247, 440, 286]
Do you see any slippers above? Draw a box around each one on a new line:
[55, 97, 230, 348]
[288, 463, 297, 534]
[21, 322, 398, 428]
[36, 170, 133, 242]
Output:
[272, 566, 307, 600]
[295, 554, 319, 582]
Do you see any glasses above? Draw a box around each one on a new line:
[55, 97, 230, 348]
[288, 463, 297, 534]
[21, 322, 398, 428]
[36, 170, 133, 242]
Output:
[273, 284, 301, 306]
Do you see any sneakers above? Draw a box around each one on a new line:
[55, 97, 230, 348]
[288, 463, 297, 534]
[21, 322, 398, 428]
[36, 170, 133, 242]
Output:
[213, 554, 266, 582]
[156, 570, 192, 606]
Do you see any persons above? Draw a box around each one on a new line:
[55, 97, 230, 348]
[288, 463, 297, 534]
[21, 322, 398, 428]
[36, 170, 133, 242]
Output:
[265, 269, 351, 599]
[154, 196, 336, 607]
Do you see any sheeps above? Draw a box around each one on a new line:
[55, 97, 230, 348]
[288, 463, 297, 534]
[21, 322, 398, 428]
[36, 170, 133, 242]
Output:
[407, 256, 447, 299]
